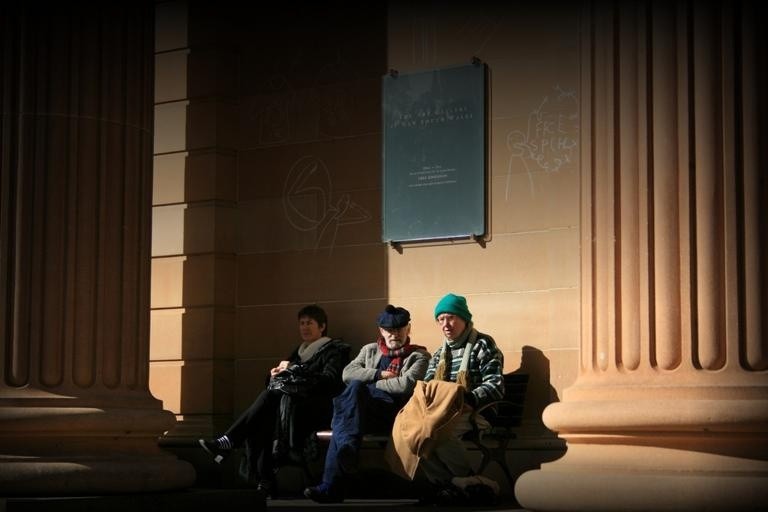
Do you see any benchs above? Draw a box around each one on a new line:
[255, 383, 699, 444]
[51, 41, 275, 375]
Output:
[311, 369, 533, 512]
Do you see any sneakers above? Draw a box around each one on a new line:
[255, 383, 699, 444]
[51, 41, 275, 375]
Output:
[336, 444, 357, 480]
[304, 485, 344, 503]
[256, 481, 277, 492]
[198, 434, 232, 465]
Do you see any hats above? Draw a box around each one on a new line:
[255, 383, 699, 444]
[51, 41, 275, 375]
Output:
[435, 294, 472, 322]
[376, 305, 411, 328]
